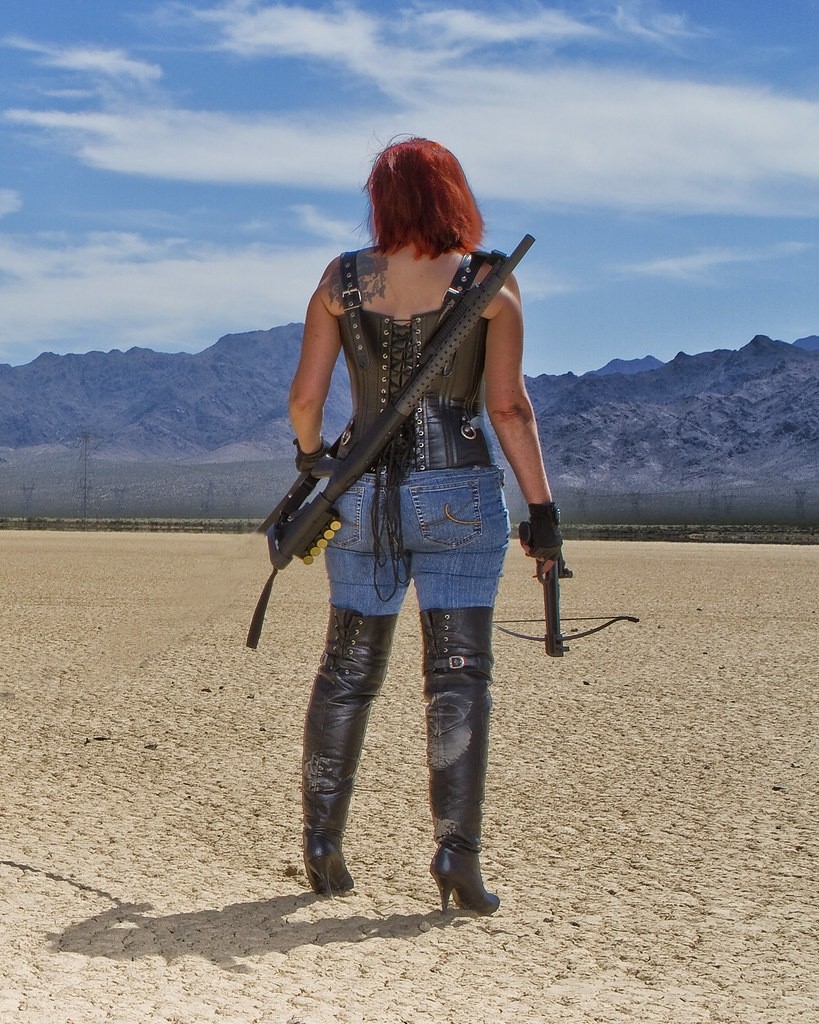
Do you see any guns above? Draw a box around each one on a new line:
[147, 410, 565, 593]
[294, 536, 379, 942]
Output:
[245, 231, 539, 571]
[517, 518, 572, 658]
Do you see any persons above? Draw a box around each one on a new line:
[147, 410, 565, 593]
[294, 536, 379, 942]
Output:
[287, 135, 564, 918]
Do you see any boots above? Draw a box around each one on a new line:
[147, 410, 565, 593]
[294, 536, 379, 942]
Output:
[303, 603, 398, 900]
[419, 606, 500, 916]
